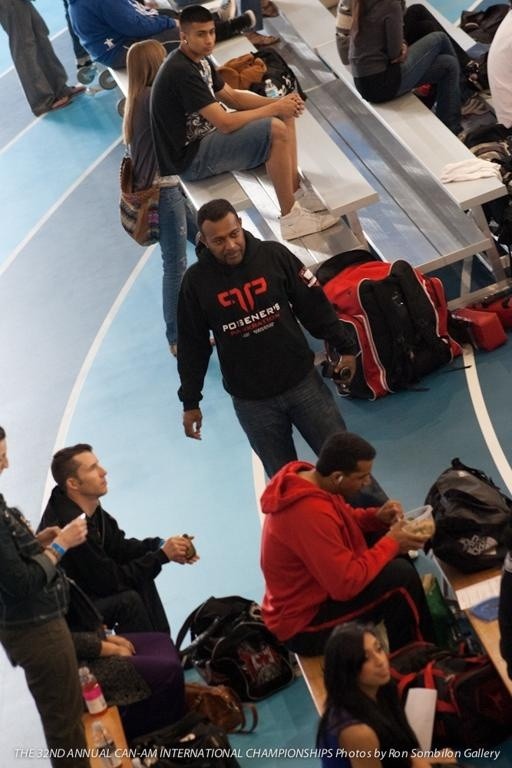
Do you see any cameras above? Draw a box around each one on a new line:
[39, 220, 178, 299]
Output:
[320, 360, 351, 382]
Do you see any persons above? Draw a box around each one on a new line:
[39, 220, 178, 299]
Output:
[0, 1, 86, 117]
[260, 432, 432, 657]
[175, 198, 356, 480]
[62, 0, 257, 69]
[315, 623, 457, 767]
[9, 506, 183, 737]
[486, 9, 512, 131]
[497, 525, 512, 681]
[348, 0, 462, 136]
[121, 39, 215, 355]
[0, 425, 92, 768]
[150, 5, 340, 240]
[35, 443, 200, 631]
[335, 0, 353, 65]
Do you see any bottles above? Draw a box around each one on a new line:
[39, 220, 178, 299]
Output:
[90, 720, 124, 768]
[264, 78, 281, 99]
[462, 630, 484, 657]
[77, 665, 109, 719]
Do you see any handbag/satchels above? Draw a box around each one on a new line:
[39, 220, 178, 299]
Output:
[175, 596, 297, 701]
[382, 640, 458, 717]
[313, 250, 462, 402]
[186, 682, 258, 734]
[248, 48, 307, 107]
[423, 456, 512, 575]
[118, 155, 159, 245]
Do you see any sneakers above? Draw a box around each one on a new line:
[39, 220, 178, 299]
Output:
[218, 0, 238, 21]
[36, 95, 70, 113]
[294, 181, 329, 214]
[278, 201, 340, 241]
[248, 0, 263, 30]
[64, 86, 86, 94]
[462, 98, 490, 115]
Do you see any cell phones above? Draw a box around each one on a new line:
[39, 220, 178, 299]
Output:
[180, 533, 196, 560]
[60, 513, 86, 534]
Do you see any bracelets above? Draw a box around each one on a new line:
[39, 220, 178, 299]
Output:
[46, 542, 65, 561]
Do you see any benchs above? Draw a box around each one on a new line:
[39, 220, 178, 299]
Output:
[273, 0, 511, 315]
[210, 20, 491, 276]
[452, 568, 512, 693]
[291, 652, 330, 712]
[106, 45, 365, 274]
